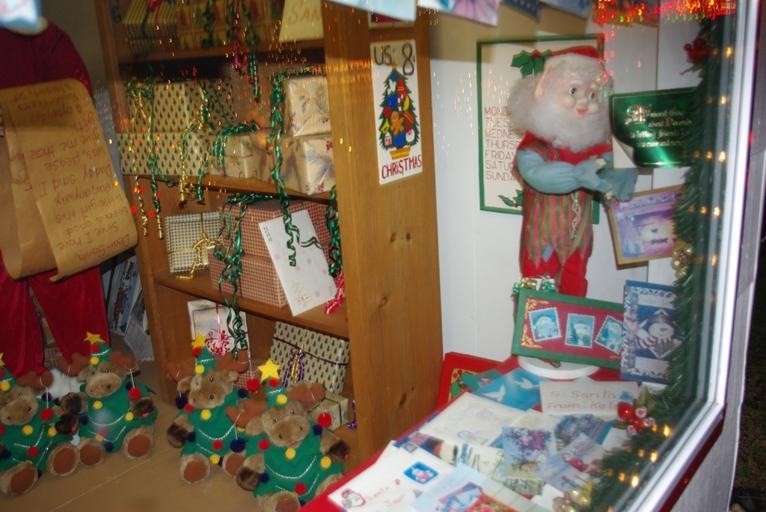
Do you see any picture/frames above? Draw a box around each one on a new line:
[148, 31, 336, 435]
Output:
[511, 288, 623, 369]
[607, 184, 684, 265]
[476, 33, 606, 225]
[620, 280, 682, 384]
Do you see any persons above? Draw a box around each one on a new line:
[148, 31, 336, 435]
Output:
[501, 38, 639, 297]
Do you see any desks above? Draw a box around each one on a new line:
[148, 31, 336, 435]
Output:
[294, 356, 625, 512]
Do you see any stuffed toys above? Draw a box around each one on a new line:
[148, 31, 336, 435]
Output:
[0, 355, 80, 502]
[164, 333, 247, 485]
[226, 356, 347, 512]
[58, 331, 158, 466]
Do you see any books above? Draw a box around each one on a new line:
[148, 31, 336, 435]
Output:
[327, 365, 640, 512]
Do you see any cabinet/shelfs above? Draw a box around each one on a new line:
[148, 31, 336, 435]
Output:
[96, 0, 444, 475]
